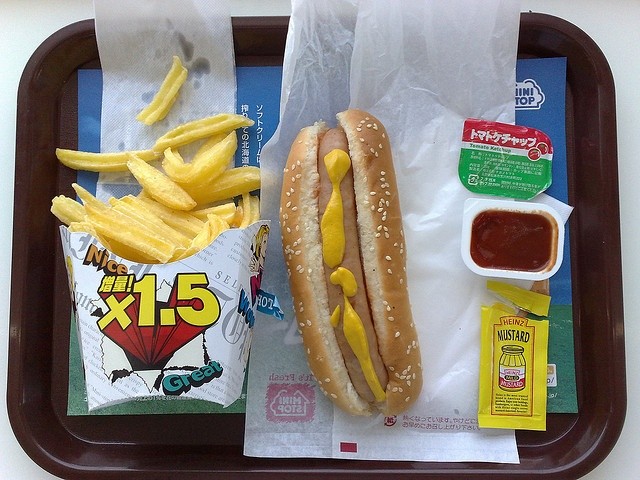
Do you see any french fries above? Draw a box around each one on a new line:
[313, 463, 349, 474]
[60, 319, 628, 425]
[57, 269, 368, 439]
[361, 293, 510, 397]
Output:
[48, 53, 272, 265]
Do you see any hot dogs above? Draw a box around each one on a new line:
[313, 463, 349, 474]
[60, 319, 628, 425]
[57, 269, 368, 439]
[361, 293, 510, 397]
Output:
[278, 109, 423, 418]
